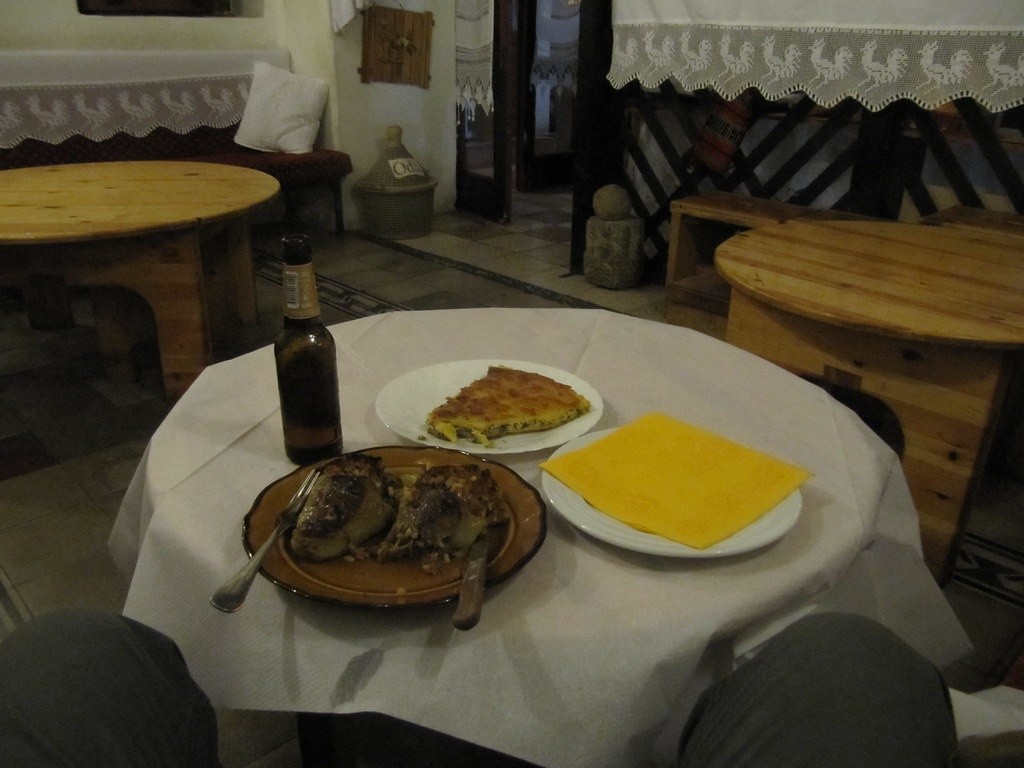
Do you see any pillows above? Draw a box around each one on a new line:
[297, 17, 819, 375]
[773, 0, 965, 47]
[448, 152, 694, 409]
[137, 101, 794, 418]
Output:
[231, 55, 329, 157]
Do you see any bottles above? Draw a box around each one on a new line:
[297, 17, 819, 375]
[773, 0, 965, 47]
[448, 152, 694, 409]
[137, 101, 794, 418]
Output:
[272, 243, 343, 467]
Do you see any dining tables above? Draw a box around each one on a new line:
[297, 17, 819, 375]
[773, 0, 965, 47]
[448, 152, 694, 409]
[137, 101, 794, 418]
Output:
[0, 158, 280, 407]
[109, 303, 974, 768]
[710, 214, 1024, 585]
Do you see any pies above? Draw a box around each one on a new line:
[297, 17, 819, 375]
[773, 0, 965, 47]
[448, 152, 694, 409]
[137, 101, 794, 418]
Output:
[426, 366, 588, 445]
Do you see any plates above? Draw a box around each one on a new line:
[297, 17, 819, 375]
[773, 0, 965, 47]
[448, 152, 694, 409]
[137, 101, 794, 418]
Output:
[376, 358, 604, 455]
[541, 427, 803, 559]
[241, 445, 547, 613]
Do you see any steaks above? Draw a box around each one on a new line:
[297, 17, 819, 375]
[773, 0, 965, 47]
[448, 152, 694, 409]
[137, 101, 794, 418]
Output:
[291, 453, 400, 560]
[377, 465, 512, 560]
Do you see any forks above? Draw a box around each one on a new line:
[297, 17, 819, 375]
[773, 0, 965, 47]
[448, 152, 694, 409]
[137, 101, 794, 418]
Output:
[209, 468, 321, 614]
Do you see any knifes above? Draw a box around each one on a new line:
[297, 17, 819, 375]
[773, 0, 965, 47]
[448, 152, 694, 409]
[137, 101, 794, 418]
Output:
[452, 533, 489, 632]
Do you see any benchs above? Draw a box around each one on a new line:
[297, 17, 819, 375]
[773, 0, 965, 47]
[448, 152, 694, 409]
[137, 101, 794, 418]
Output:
[0, 45, 353, 236]
[664, 190, 831, 314]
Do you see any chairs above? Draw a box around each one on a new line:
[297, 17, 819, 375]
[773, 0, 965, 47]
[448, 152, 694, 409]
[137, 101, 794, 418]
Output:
[669, 609, 964, 768]
[0, 606, 221, 766]
